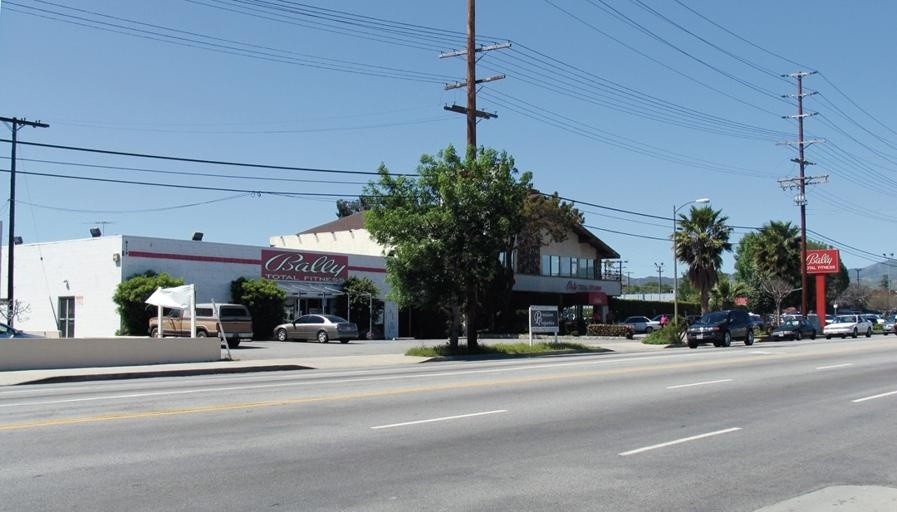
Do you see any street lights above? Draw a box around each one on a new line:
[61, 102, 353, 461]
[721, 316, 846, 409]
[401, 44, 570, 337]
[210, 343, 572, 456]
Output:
[673, 199, 711, 328]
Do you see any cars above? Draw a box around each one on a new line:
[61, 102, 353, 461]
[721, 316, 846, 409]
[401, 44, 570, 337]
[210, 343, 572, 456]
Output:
[272, 313, 358, 345]
[616, 315, 703, 334]
[751, 309, 897, 342]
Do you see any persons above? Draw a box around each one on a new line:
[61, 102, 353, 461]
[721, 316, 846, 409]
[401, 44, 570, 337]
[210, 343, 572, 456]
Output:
[659, 313, 668, 327]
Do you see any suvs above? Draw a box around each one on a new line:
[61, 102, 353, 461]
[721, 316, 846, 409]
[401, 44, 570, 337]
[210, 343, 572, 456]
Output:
[686, 310, 755, 349]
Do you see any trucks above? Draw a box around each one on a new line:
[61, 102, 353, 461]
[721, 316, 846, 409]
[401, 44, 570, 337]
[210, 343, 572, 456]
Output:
[148, 302, 254, 350]
